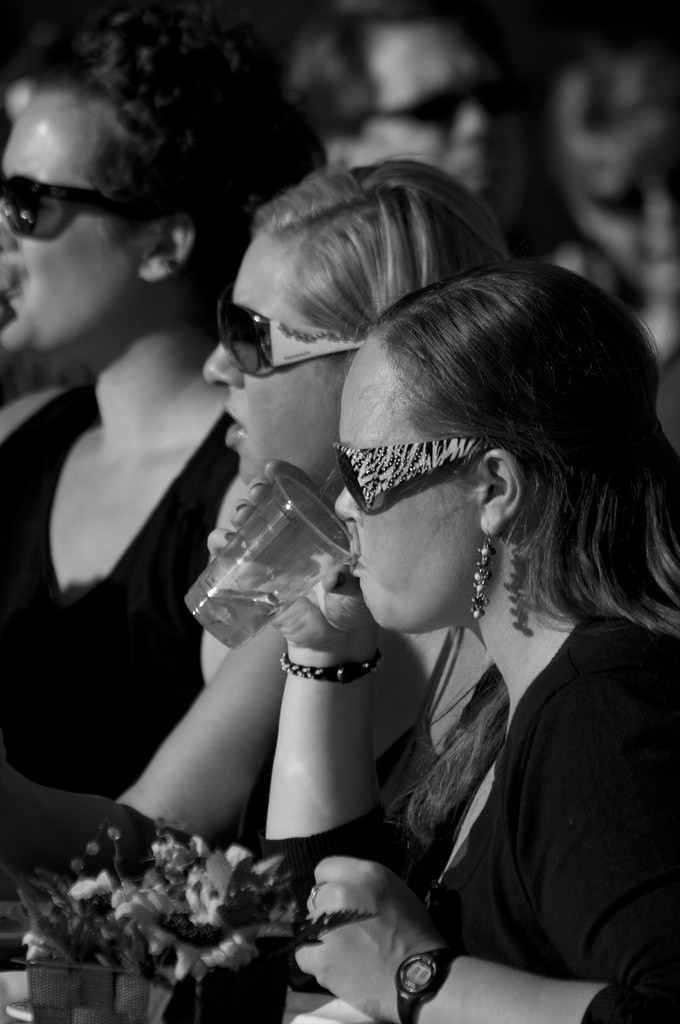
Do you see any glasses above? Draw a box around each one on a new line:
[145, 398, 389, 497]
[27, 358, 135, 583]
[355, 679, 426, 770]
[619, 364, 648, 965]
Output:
[216, 281, 363, 377]
[331, 437, 519, 513]
[0, 172, 154, 242]
[369, 76, 525, 131]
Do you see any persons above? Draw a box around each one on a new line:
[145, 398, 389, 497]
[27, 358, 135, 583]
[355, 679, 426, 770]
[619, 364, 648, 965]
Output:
[3, 0, 678, 1024]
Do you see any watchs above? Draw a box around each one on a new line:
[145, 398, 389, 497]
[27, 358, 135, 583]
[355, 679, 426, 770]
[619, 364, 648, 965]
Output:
[394, 947, 469, 1024]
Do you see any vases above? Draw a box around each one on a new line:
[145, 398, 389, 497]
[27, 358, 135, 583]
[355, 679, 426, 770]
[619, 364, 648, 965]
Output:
[152, 946, 289, 1024]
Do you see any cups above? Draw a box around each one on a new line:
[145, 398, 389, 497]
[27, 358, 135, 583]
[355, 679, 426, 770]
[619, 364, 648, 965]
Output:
[0, 204, 27, 295]
[184, 473, 356, 649]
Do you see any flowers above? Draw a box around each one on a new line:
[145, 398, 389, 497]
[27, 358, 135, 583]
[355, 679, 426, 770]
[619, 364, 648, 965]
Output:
[6, 820, 379, 979]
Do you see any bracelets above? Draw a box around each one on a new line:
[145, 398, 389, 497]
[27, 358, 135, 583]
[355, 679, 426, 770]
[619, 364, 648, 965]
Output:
[280, 647, 383, 682]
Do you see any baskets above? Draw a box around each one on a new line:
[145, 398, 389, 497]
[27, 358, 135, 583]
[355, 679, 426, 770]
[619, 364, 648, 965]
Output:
[26, 962, 149, 1024]
